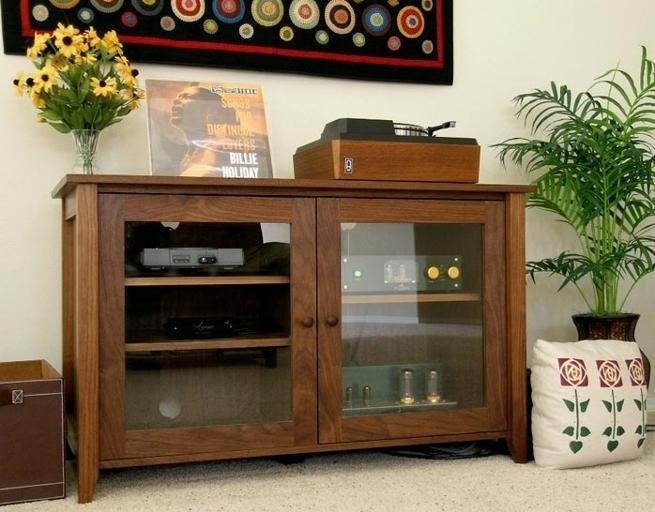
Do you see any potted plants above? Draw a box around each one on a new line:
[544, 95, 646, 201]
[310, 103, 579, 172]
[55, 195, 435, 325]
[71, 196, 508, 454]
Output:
[484, 40, 654, 396]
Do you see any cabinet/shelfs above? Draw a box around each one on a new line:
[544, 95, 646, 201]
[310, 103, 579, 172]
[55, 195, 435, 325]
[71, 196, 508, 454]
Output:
[45, 163, 547, 505]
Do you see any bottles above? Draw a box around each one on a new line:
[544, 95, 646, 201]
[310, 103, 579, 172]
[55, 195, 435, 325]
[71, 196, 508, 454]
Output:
[345, 387, 353, 406]
[362, 386, 371, 406]
[400, 368, 416, 404]
[425, 369, 441, 402]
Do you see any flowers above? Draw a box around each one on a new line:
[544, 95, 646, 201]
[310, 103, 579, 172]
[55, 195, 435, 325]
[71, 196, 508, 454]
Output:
[6, 22, 150, 157]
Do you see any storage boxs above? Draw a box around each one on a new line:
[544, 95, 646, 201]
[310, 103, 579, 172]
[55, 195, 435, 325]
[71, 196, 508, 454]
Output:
[0, 354, 71, 508]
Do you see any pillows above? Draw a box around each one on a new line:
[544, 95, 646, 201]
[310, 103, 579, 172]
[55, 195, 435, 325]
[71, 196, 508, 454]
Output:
[525, 335, 650, 472]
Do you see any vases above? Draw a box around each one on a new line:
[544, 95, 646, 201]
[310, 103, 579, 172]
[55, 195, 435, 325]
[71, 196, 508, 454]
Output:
[66, 126, 106, 177]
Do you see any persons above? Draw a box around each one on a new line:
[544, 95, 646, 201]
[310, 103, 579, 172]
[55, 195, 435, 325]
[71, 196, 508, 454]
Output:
[170, 87, 241, 177]
